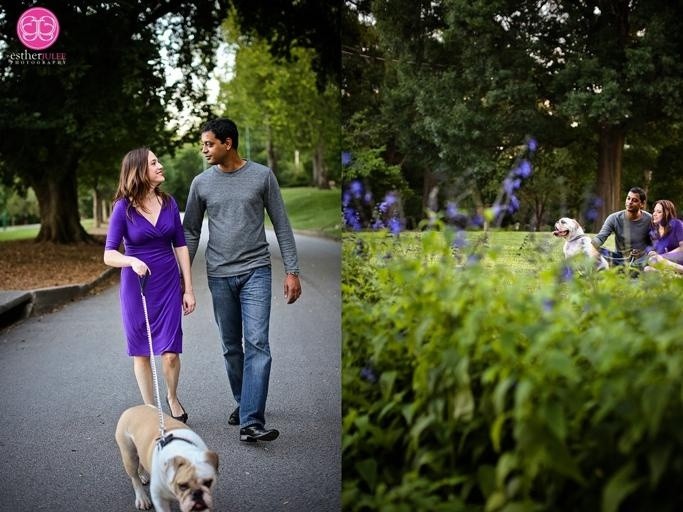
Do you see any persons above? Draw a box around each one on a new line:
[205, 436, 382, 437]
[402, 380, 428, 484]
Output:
[180, 118, 302, 442]
[103, 148, 197, 424]
[591, 186, 653, 276]
[634, 200, 683, 278]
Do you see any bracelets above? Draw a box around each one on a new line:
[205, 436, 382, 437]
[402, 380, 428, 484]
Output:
[290, 272, 300, 275]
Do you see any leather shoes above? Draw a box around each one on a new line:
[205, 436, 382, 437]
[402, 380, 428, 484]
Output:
[166, 393, 187, 423]
[227, 406, 240, 425]
[240, 423, 279, 442]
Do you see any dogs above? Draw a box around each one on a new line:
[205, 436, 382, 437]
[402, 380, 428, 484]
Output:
[552, 217, 609, 273]
[114, 404, 219, 512]
